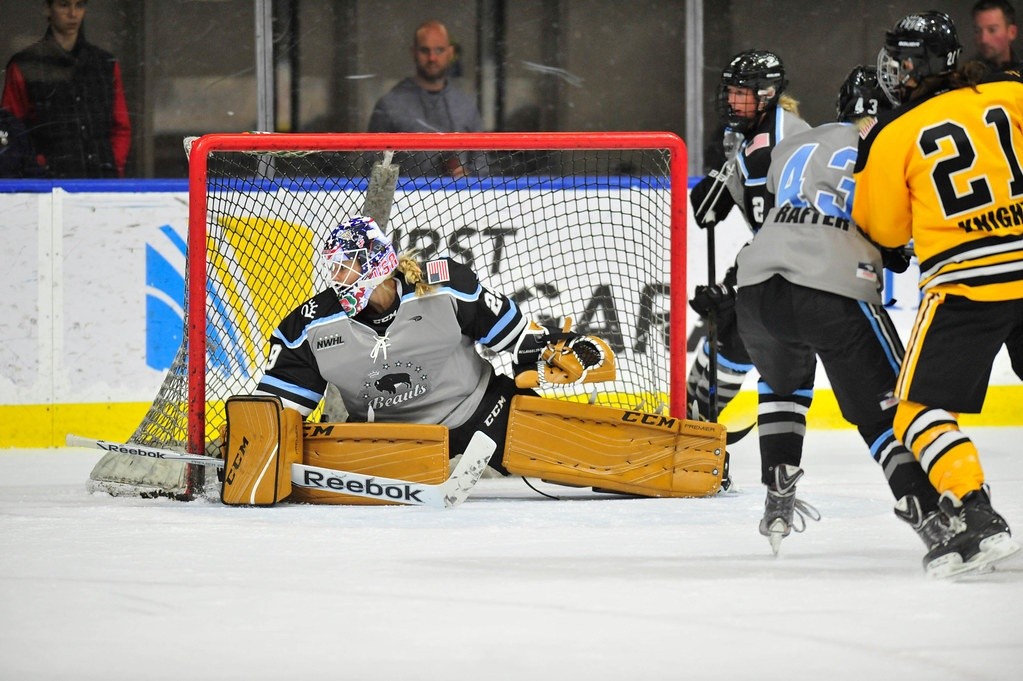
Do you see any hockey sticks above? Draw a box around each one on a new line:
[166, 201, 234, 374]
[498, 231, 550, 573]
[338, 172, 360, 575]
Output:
[704, 220, 732, 495]
[62, 368, 518, 506]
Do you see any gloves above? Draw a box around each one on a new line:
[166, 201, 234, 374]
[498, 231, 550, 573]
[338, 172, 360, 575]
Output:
[689, 282, 737, 324]
[879, 242, 915, 273]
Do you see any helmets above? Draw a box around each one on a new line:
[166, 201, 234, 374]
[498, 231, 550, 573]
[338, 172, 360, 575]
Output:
[714, 51, 788, 132]
[321, 214, 399, 318]
[834, 64, 895, 124]
[876, 10, 963, 107]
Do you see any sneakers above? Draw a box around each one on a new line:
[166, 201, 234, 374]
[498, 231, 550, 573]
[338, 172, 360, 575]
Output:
[895, 495, 958, 551]
[922, 484, 1023, 581]
[758, 464, 821, 557]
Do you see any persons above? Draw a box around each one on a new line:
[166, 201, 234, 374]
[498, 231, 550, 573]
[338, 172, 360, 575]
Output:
[735, 63, 951, 557]
[848, 9, 1023, 579]
[974, 0, 1017, 61]
[252, 214, 616, 494]
[363, 20, 490, 177]
[0, 0, 133, 179]
[686, 50, 812, 494]
